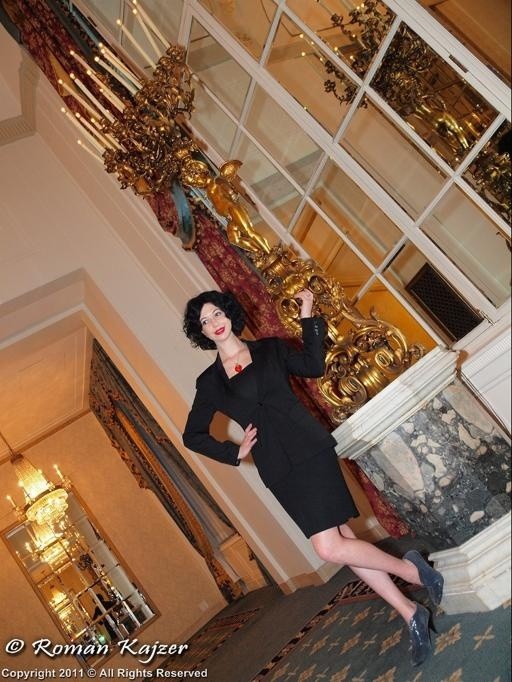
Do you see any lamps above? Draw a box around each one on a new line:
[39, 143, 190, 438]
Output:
[36, 570, 76, 621]
[0, 432, 71, 528]
[24, 520, 86, 576]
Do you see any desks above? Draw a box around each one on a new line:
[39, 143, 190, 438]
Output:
[117, 602, 147, 633]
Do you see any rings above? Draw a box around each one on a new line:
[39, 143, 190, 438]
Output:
[306, 294, 311, 297]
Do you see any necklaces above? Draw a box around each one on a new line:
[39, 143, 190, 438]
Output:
[221, 339, 243, 373]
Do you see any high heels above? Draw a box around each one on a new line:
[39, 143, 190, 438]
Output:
[406, 600, 441, 667]
[401, 549, 444, 606]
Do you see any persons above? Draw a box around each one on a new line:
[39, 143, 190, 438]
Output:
[90, 592, 121, 644]
[181, 286, 445, 667]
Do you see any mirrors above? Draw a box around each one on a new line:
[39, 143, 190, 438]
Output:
[1, 485, 162, 678]
